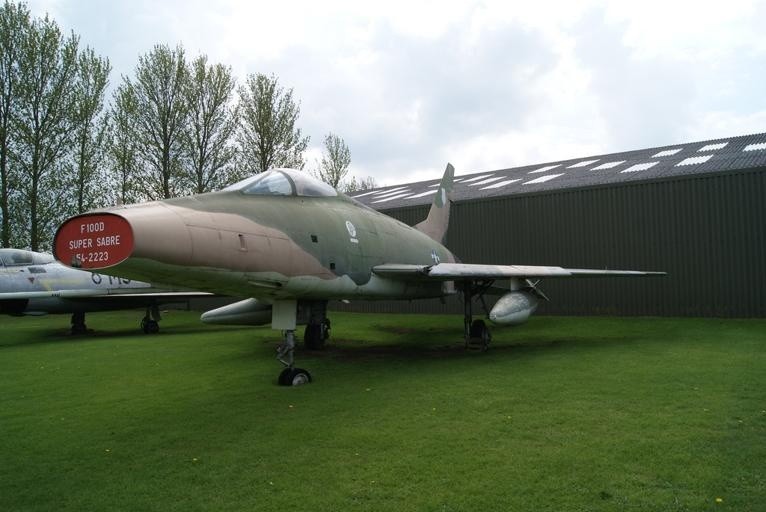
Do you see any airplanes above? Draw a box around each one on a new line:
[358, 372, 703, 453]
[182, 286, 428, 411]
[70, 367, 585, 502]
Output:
[50, 161, 673, 389]
[1, 246, 216, 333]
